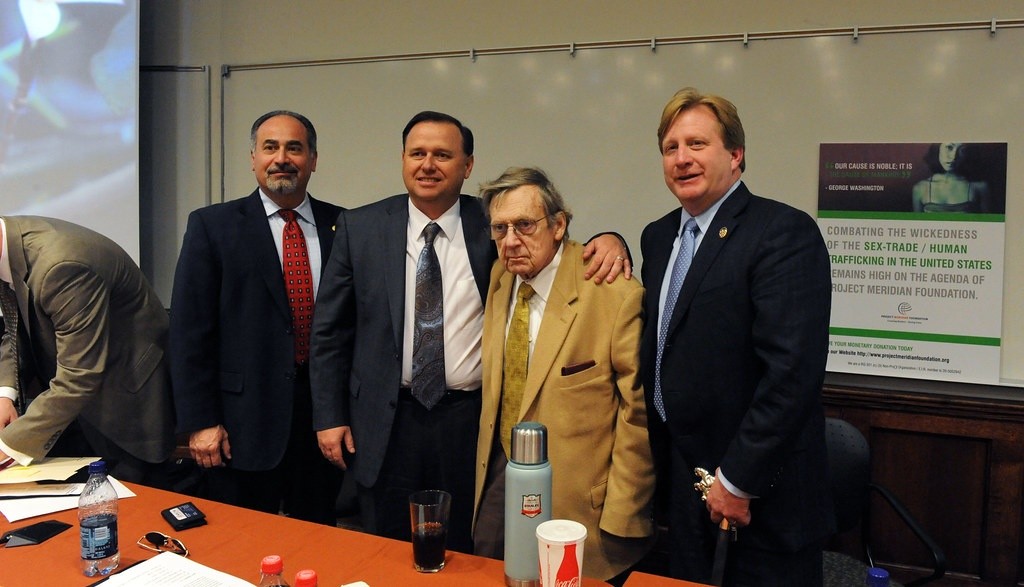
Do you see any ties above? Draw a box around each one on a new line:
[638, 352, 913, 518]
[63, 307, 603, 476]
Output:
[278, 209, 315, 365]
[500, 281, 536, 462]
[653, 217, 698, 422]
[0, 279, 26, 416]
[412, 223, 446, 411]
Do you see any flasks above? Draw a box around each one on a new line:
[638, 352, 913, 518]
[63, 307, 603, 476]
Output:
[502, 421, 553, 587]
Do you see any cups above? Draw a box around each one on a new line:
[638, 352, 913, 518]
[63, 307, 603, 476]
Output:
[410, 490, 451, 572]
[535, 519, 587, 587]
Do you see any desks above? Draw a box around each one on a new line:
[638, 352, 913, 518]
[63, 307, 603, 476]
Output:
[0, 449, 731, 587]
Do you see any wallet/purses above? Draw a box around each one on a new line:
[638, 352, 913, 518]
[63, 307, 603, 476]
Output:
[161, 502, 207, 532]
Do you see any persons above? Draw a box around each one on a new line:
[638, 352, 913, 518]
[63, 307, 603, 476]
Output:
[167, 110, 351, 526]
[0, 214, 177, 486]
[911, 143, 991, 214]
[467, 162, 663, 586]
[307, 110, 632, 547]
[630, 87, 834, 587]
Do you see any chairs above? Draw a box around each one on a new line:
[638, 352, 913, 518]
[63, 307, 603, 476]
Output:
[816, 412, 954, 587]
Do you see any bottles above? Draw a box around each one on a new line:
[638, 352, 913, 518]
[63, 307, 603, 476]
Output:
[77, 460, 120, 578]
[258, 555, 291, 587]
[293, 569, 318, 587]
[864, 568, 890, 587]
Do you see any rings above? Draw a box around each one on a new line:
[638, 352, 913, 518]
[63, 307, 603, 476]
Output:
[617, 258, 624, 262]
[719, 517, 730, 534]
[728, 520, 737, 525]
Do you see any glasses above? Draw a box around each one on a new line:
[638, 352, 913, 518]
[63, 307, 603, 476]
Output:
[486, 215, 548, 240]
[137, 531, 189, 558]
